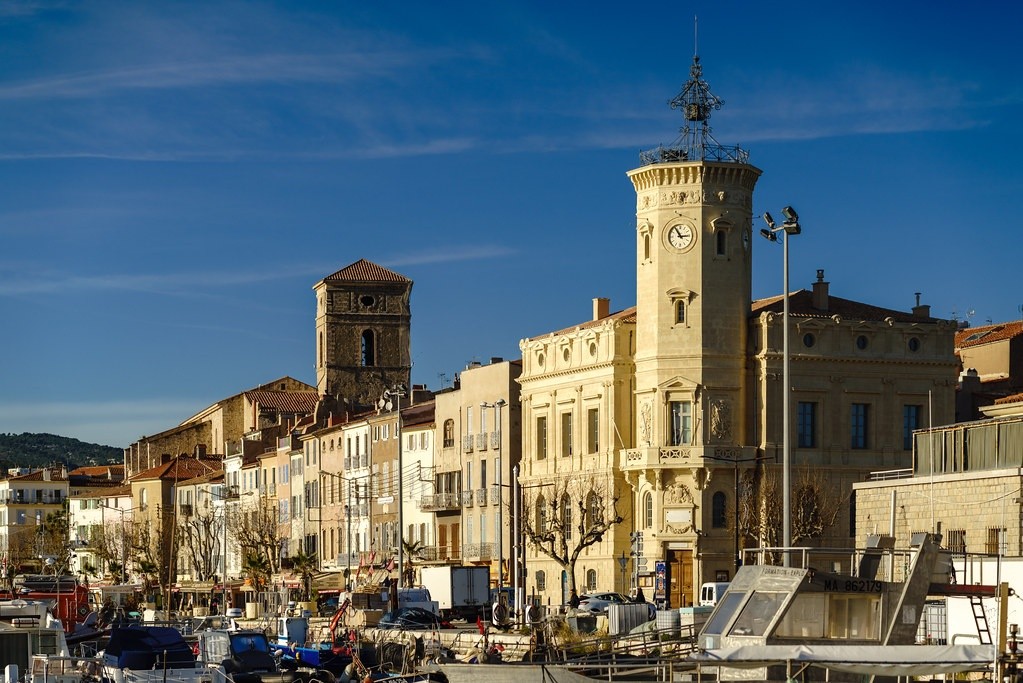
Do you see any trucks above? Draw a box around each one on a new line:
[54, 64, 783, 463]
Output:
[413, 566, 490, 624]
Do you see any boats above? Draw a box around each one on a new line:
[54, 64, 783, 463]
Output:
[0, 574, 399, 683]
[433, 533, 1022, 683]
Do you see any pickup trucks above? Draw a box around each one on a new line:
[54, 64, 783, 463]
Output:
[394, 587, 439, 617]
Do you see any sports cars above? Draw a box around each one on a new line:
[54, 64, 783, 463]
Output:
[563, 593, 657, 621]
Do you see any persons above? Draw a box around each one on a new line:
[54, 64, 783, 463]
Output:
[568, 589, 580, 609]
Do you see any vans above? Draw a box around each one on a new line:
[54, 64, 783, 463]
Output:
[491, 587, 515, 611]
[700, 582, 782, 617]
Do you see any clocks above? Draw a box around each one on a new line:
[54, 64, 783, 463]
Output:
[669, 225, 691, 249]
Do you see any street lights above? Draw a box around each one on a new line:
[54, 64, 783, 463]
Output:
[480, 399, 507, 598]
[21, 512, 68, 575]
[512, 466, 519, 625]
[318, 470, 382, 589]
[759, 204, 801, 566]
[199, 487, 254, 616]
[98, 504, 147, 584]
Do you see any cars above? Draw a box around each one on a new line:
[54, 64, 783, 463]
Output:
[317, 596, 339, 616]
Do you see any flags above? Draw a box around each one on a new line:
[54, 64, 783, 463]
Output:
[356, 552, 395, 579]
